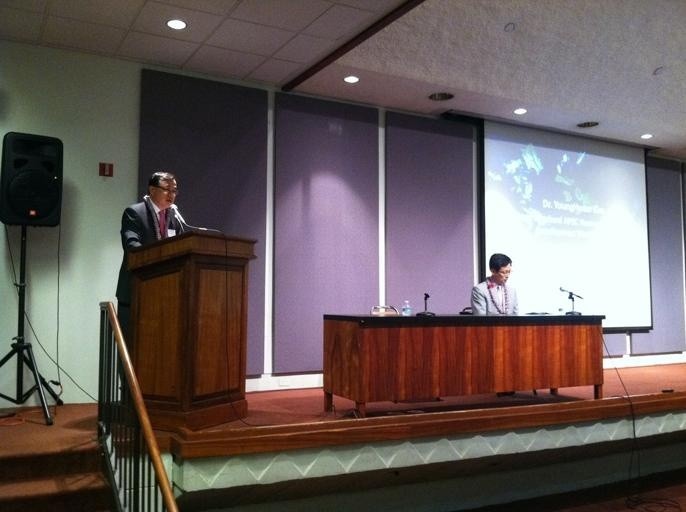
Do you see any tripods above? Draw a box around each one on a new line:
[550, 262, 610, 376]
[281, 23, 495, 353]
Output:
[0, 225, 64, 425]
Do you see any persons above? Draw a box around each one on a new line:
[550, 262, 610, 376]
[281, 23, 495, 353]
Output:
[471, 253, 518, 316]
[115, 171, 183, 405]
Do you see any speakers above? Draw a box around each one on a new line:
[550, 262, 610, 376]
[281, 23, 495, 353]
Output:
[0, 132, 63, 226]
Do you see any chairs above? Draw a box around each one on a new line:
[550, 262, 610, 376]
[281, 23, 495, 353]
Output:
[369, 304, 400, 316]
[458, 307, 471, 315]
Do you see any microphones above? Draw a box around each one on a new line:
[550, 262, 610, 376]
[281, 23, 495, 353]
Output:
[170, 203, 185, 225]
[560, 286, 583, 299]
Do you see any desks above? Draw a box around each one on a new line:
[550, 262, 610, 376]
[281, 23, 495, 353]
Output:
[319, 309, 607, 420]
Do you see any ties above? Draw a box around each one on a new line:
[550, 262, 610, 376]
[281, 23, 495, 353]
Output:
[497, 286, 503, 310]
[159, 210, 165, 238]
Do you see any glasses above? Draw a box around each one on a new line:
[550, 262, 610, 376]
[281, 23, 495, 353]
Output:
[157, 186, 178, 196]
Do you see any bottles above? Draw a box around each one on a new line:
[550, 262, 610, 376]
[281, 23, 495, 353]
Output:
[401, 300, 413, 316]
